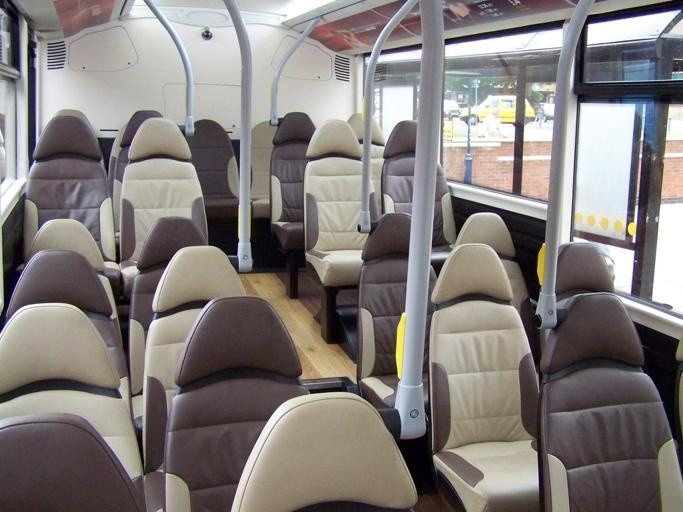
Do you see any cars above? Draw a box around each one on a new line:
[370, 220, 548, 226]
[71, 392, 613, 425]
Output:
[443, 96, 555, 126]
